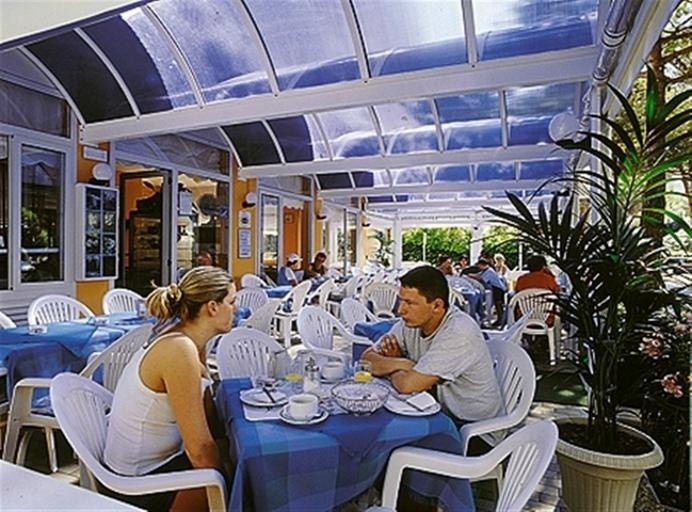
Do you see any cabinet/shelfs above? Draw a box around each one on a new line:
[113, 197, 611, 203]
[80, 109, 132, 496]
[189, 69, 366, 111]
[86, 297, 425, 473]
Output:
[74, 182, 119, 282]
[128, 209, 198, 297]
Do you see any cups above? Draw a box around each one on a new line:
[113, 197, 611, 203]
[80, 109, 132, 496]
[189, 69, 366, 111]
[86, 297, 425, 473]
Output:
[322, 362, 345, 380]
[354, 360, 373, 381]
[288, 393, 319, 421]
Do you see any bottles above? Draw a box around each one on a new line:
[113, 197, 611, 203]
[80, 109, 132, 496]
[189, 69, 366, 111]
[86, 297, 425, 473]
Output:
[302, 364, 322, 392]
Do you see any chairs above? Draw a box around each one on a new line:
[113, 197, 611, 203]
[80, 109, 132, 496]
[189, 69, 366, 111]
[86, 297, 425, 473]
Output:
[460, 338, 537, 501]
[2, 322, 153, 473]
[49, 372, 229, 512]
[369, 418, 559, 511]
[1, 259, 564, 379]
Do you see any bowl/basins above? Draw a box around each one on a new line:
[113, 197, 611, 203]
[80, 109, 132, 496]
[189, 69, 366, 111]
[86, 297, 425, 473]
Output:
[331, 383, 391, 417]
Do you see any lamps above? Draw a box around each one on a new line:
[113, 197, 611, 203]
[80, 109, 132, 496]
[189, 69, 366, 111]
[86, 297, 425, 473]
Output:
[242, 192, 257, 208]
[89, 164, 113, 185]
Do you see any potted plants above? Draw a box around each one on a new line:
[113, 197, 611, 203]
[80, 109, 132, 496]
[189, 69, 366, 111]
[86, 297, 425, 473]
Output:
[463, 60, 692, 511]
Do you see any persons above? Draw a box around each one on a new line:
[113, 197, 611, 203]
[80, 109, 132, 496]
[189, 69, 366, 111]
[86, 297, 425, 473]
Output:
[307, 252, 328, 278]
[358, 261, 511, 511]
[274, 252, 305, 288]
[433, 250, 572, 359]
[96, 262, 240, 511]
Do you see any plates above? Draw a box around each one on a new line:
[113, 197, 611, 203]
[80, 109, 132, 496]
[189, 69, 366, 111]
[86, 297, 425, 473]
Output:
[385, 392, 442, 418]
[278, 405, 328, 426]
[238, 386, 291, 407]
[320, 379, 347, 383]
